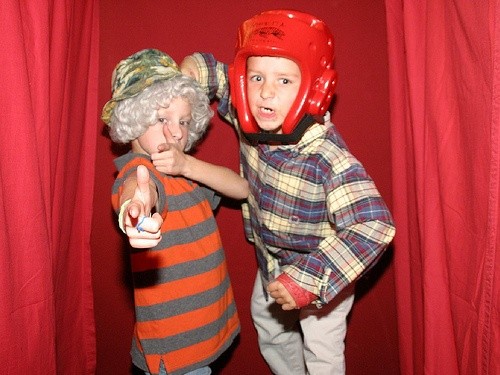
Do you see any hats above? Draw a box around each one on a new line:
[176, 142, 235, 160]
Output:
[101, 48, 184, 124]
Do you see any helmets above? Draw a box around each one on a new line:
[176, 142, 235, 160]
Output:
[228, 10, 338, 144]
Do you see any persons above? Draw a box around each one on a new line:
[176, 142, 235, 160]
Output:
[102, 48, 249, 375]
[180, 9, 396, 375]
